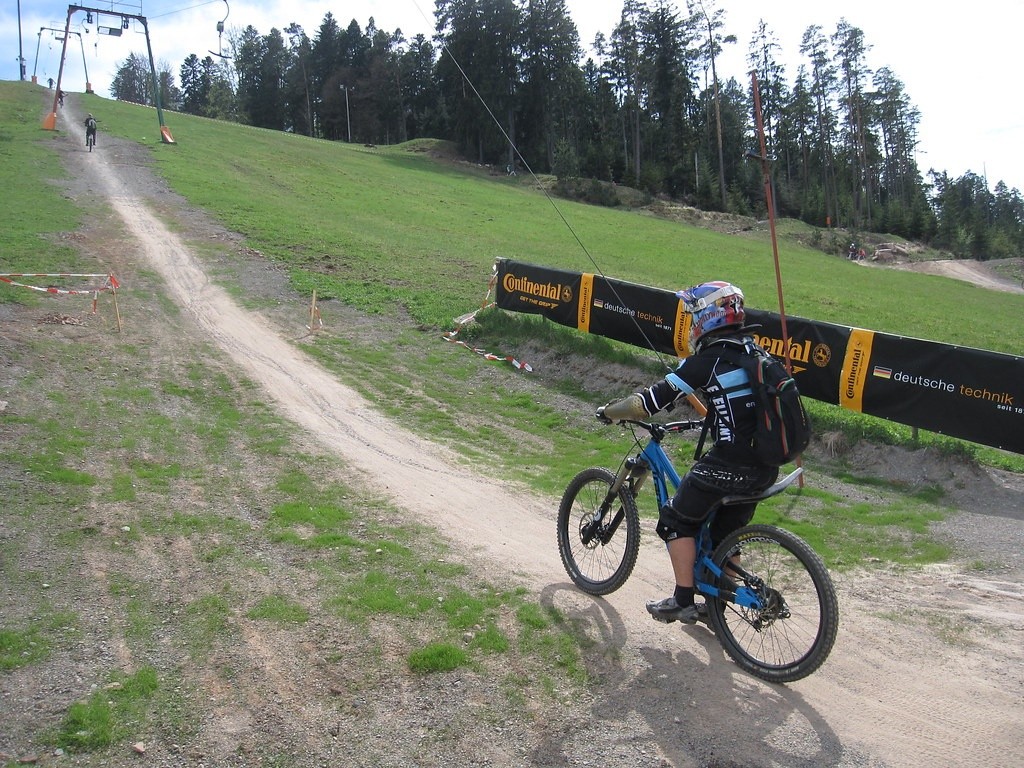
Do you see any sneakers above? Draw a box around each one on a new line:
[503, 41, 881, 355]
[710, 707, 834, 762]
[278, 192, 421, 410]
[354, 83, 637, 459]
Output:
[695, 602, 727, 632]
[645, 597, 699, 625]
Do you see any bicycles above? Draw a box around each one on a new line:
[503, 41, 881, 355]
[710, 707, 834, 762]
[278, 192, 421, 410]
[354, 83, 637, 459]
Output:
[56, 94, 66, 110]
[84, 123, 96, 152]
[556, 412, 839, 684]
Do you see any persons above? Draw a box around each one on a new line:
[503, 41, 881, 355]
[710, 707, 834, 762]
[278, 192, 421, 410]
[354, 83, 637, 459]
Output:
[859, 248, 865, 256]
[47, 78, 56, 87]
[596, 281, 779, 631]
[58, 88, 66, 106]
[84, 113, 97, 146]
[847, 243, 856, 260]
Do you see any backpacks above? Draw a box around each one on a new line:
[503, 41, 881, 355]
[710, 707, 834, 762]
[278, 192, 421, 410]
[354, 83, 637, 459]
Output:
[88, 118, 96, 130]
[701, 341, 811, 468]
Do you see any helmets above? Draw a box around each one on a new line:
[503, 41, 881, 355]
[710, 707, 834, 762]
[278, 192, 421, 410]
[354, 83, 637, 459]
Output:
[676, 281, 746, 352]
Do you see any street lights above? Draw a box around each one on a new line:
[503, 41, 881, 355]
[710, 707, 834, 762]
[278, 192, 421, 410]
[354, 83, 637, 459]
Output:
[341, 84, 355, 142]
